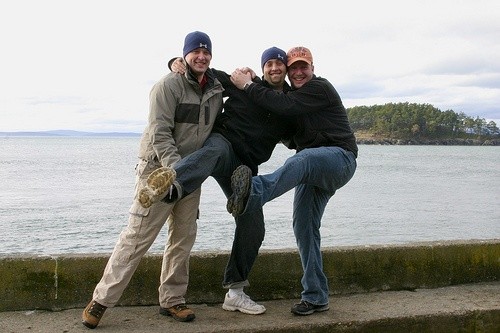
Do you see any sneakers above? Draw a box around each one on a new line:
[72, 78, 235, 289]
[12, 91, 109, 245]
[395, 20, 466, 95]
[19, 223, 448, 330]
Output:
[290, 299, 330, 315]
[221, 289, 267, 315]
[82, 298, 108, 329]
[226, 164, 253, 218]
[138, 166, 178, 208]
[159, 302, 196, 322]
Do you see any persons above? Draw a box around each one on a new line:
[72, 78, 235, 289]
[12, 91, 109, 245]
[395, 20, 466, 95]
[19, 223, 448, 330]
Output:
[80, 31, 224, 330]
[227, 46, 358, 316]
[138, 47, 296, 315]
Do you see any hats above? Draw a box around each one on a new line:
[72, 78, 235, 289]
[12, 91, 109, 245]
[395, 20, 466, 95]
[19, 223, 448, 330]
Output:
[183, 31, 212, 58]
[286, 46, 313, 67]
[261, 46, 288, 75]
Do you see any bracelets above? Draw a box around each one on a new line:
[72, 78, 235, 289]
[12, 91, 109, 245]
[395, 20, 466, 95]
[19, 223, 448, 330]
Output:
[243, 81, 254, 93]
